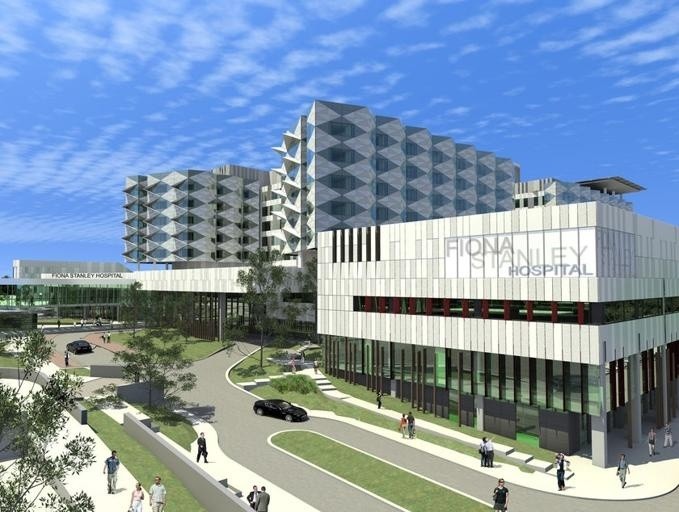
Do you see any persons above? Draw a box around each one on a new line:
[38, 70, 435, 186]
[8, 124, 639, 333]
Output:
[376, 391, 384, 409]
[254, 486, 270, 511]
[400, 412, 408, 440]
[64, 350, 69, 367]
[493, 478, 510, 512]
[196, 432, 209, 463]
[148, 476, 166, 512]
[106, 331, 112, 344]
[99, 332, 107, 344]
[103, 450, 121, 494]
[478, 441, 487, 467]
[291, 357, 296, 374]
[482, 435, 498, 468]
[663, 422, 673, 448]
[648, 426, 656, 456]
[618, 453, 631, 489]
[57, 319, 61, 329]
[129, 482, 145, 511]
[41, 324, 45, 334]
[301, 350, 305, 362]
[406, 412, 416, 439]
[247, 485, 263, 509]
[313, 358, 320, 374]
[555, 452, 570, 491]
[81, 318, 85, 329]
